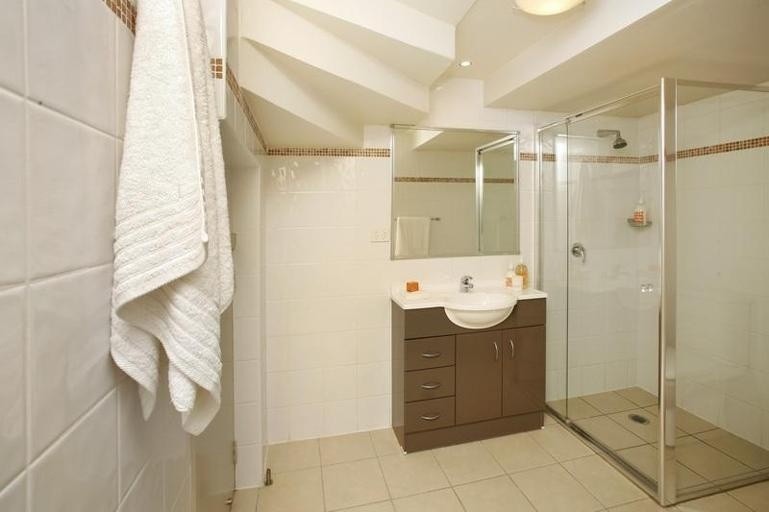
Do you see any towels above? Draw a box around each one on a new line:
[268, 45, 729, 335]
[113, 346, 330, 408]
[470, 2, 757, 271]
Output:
[111, 0, 235, 436]
[395, 217, 430, 259]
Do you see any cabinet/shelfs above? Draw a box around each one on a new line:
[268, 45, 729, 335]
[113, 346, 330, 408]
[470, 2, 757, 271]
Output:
[392, 297, 546, 453]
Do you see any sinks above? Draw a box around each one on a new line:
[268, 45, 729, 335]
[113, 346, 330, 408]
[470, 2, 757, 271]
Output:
[444, 292, 518, 330]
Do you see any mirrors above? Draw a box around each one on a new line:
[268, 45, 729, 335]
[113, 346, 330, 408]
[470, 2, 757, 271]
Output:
[391, 126, 520, 259]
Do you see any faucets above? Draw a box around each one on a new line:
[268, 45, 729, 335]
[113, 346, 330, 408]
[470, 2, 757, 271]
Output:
[460, 275, 473, 293]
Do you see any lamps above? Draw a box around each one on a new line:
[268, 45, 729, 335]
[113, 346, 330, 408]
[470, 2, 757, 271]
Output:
[516, 0, 585, 16]
